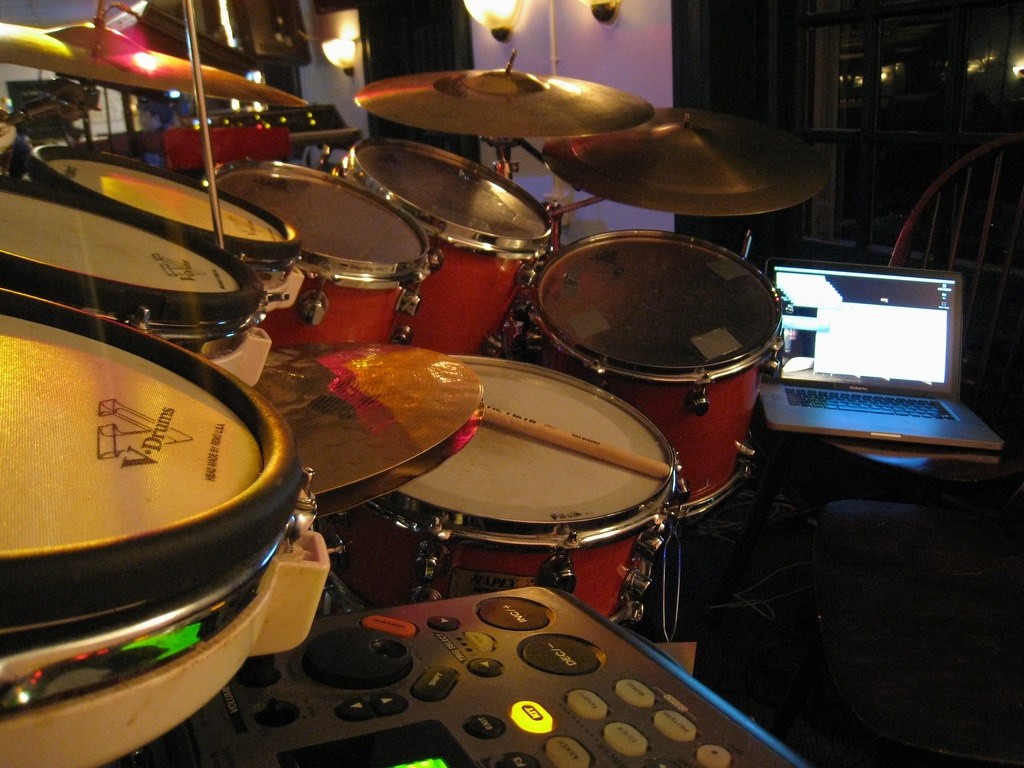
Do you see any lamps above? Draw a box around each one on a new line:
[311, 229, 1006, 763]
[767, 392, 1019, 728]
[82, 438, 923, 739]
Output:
[320, 40, 355, 76]
[461, 0, 522, 43]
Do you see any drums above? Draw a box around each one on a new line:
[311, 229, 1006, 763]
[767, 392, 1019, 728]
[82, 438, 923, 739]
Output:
[516, 222, 793, 537]
[305, 345, 695, 652]
[200, 129, 560, 363]
[0, 131, 320, 768]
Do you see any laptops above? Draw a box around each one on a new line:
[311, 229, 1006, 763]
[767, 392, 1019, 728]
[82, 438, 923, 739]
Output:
[758, 255, 1005, 450]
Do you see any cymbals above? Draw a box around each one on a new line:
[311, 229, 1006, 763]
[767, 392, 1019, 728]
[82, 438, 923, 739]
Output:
[532, 96, 843, 222]
[342, 61, 664, 145]
[249, 331, 495, 529]
[0, 10, 316, 115]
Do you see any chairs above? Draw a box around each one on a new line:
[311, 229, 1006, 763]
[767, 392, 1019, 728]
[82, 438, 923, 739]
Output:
[763, 497, 1024, 768]
[704, 130, 1024, 623]
